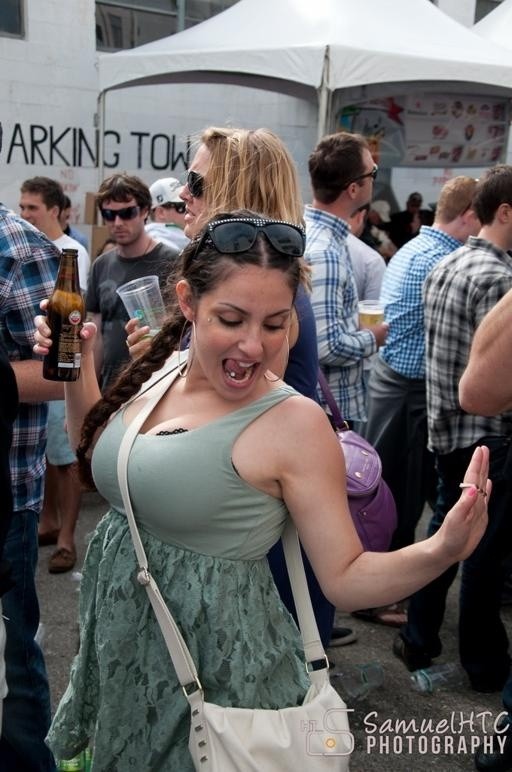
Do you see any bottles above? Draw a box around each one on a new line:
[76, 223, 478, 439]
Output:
[42, 247, 86, 383]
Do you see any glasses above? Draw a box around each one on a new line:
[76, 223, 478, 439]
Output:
[188, 171, 204, 199]
[192, 216, 307, 265]
[344, 164, 378, 191]
[100, 205, 141, 220]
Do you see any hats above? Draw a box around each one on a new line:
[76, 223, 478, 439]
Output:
[149, 178, 185, 208]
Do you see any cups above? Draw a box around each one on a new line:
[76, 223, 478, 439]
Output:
[340, 661, 385, 703]
[116, 275, 166, 342]
[357, 299, 386, 330]
[409, 661, 461, 694]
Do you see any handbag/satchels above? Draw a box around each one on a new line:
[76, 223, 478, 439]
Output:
[335, 427, 397, 552]
[187, 670, 350, 772]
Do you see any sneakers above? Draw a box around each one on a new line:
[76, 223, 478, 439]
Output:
[39, 527, 59, 545]
[326, 627, 356, 647]
[50, 545, 78, 573]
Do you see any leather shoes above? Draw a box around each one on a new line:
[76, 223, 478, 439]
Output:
[393, 631, 430, 675]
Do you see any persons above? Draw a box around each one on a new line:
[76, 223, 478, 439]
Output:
[0, 122, 512, 772]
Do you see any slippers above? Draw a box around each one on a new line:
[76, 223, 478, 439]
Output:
[348, 600, 407, 626]
